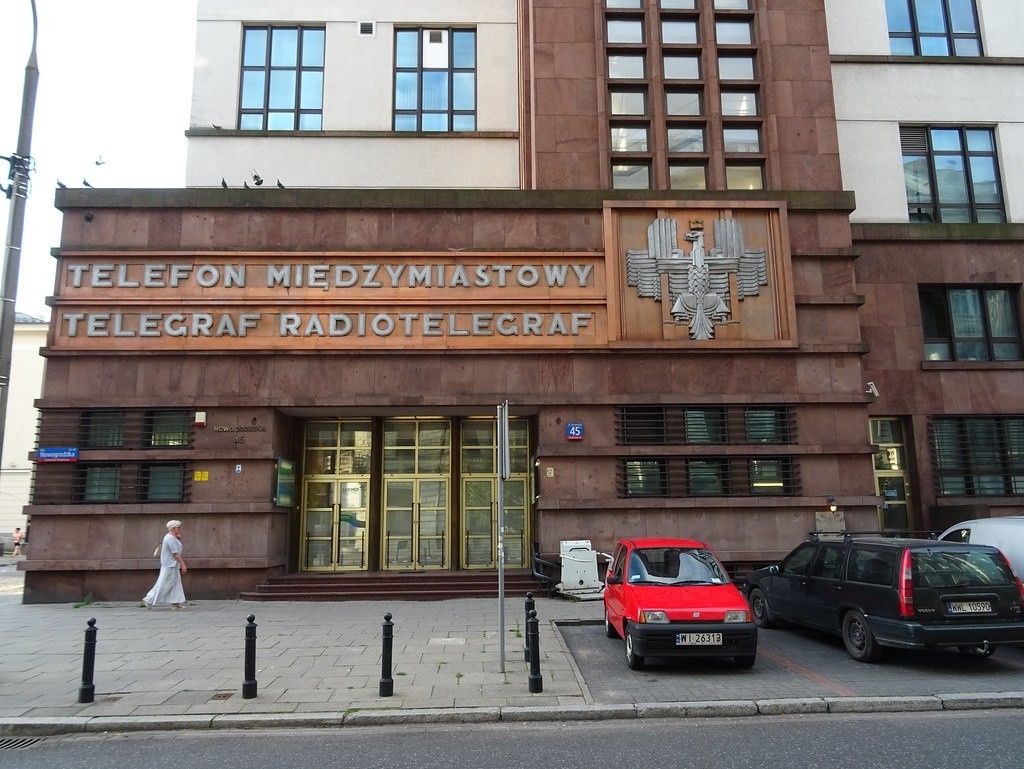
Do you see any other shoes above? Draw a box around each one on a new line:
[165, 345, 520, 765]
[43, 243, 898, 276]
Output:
[171, 605, 187, 611]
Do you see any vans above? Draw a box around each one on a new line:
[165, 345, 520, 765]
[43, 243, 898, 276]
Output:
[932, 515, 1024, 590]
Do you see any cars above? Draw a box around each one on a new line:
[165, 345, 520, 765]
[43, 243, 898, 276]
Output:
[602, 537, 758, 672]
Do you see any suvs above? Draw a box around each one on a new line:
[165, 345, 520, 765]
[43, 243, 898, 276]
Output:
[743, 529, 1024, 664]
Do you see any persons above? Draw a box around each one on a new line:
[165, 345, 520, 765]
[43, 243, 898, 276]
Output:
[142, 519, 187, 611]
[11, 527, 24, 557]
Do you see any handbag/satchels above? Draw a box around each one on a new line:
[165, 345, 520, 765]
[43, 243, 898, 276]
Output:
[153, 543, 163, 558]
